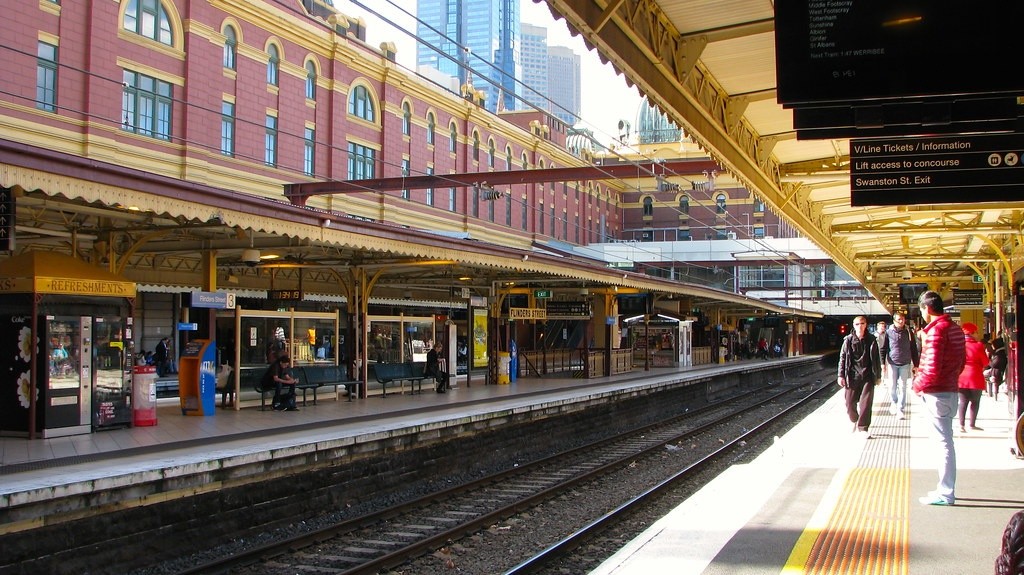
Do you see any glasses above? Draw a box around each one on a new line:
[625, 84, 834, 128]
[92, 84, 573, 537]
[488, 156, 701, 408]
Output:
[855, 323, 866, 326]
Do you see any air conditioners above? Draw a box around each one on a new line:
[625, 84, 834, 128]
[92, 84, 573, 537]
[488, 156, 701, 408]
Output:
[727, 233, 736, 240]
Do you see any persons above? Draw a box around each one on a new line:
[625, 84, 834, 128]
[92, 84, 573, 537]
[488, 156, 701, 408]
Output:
[958, 322, 989, 432]
[837, 316, 882, 439]
[374, 328, 392, 363]
[878, 311, 920, 420]
[307, 325, 316, 345]
[275, 321, 286, 351]
[740, 338, 783, 360]
[261, 356, 298, 410]
[423, 337, 434, 351]
[911, 290, 967, 506]
[316, 335, 345, 365]
[873, 322, 1007, 395]
[135, 337, 173, 377]
[266, 342, 284, 364]
[427, 342, 450, 394]
[458, 340, 468, 365]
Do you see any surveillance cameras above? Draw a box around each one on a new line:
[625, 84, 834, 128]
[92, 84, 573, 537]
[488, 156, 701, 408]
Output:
[242, 249, 260, 267]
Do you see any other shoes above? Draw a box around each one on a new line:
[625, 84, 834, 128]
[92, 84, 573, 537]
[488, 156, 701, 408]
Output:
[919, 490, 956, 506]
[850, 421, 872, 440]
[890, 402, 906, 420]
[437, 387, 446, 393]
[960, 424, 984, 433]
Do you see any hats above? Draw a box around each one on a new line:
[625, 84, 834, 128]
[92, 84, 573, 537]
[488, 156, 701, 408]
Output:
[961, 322, 979, 334]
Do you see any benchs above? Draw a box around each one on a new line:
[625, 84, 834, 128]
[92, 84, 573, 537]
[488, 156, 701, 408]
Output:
[216, 364, 363, 411]
[367, 361, 456, 398]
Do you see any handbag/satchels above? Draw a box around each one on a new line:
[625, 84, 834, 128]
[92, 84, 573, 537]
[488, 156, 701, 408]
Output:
[272, 393, 297, 410]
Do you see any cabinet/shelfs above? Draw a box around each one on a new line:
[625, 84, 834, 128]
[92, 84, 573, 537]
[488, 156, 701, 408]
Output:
[49, 332, 80, 383]
